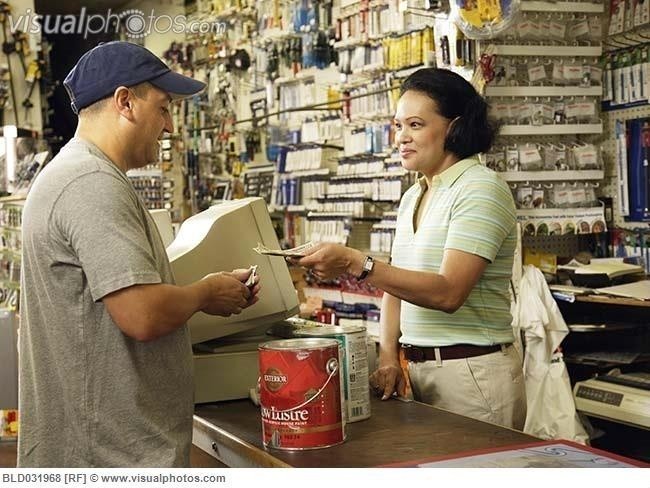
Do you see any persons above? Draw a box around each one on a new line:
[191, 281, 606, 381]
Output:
[284, 67, 527, 434]
[17, 40, 261, 467]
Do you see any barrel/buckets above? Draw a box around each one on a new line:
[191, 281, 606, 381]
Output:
[250, 337, 343, 451]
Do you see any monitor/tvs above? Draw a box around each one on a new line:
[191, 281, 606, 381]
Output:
[164, 197, 301, 354]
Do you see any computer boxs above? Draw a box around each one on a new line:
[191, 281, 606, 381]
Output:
[192, 334, 286, 404]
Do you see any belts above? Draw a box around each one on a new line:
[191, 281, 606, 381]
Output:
[401, 343, 514, 362]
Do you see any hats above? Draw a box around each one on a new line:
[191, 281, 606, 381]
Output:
[62, 40, 207, 114]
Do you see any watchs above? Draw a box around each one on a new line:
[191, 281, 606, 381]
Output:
[357, 256, 373, 283]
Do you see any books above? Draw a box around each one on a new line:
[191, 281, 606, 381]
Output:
[574, 260, 646, 278]
